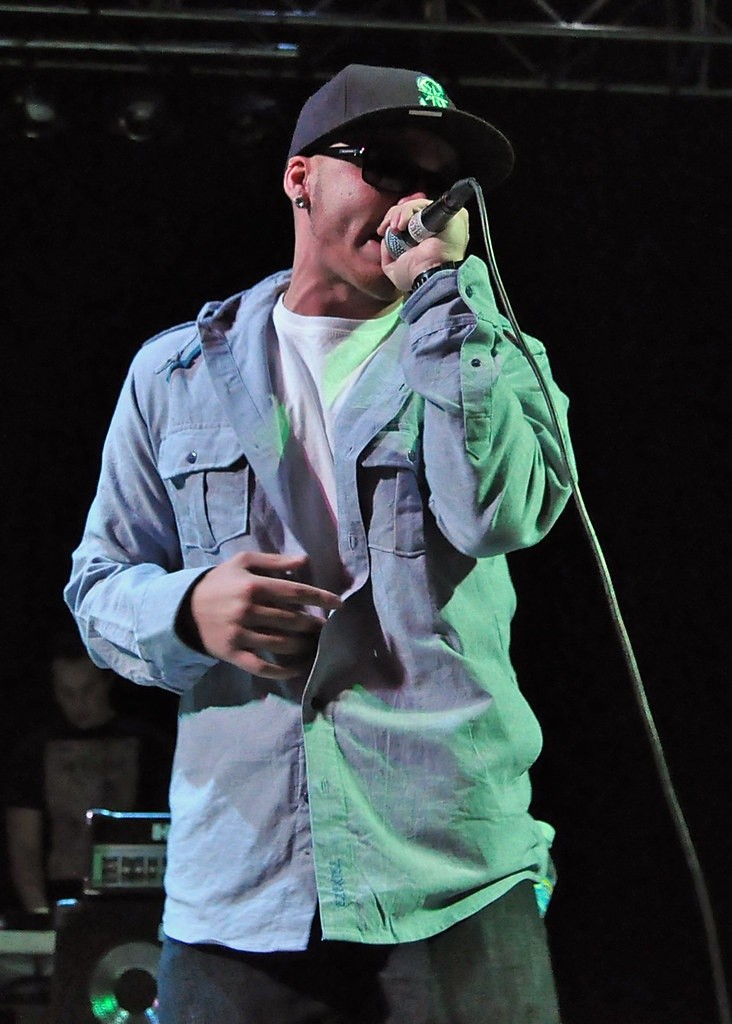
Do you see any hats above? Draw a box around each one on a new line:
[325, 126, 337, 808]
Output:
[287, 63, 515, 198]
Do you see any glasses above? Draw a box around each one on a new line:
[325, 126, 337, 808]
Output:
[318, 135, 457, 202]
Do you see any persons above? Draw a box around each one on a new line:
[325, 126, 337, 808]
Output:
[5, 644, 174, 916]
[62, 66, 581, 1024]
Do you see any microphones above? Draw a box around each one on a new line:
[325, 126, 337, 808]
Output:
[384, 177, 477, 262]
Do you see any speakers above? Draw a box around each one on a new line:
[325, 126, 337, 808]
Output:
[51, 891, 169, 1024]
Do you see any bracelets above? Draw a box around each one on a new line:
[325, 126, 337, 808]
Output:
[31, 906, 53, 915]
[408, 259, 466, 295]
